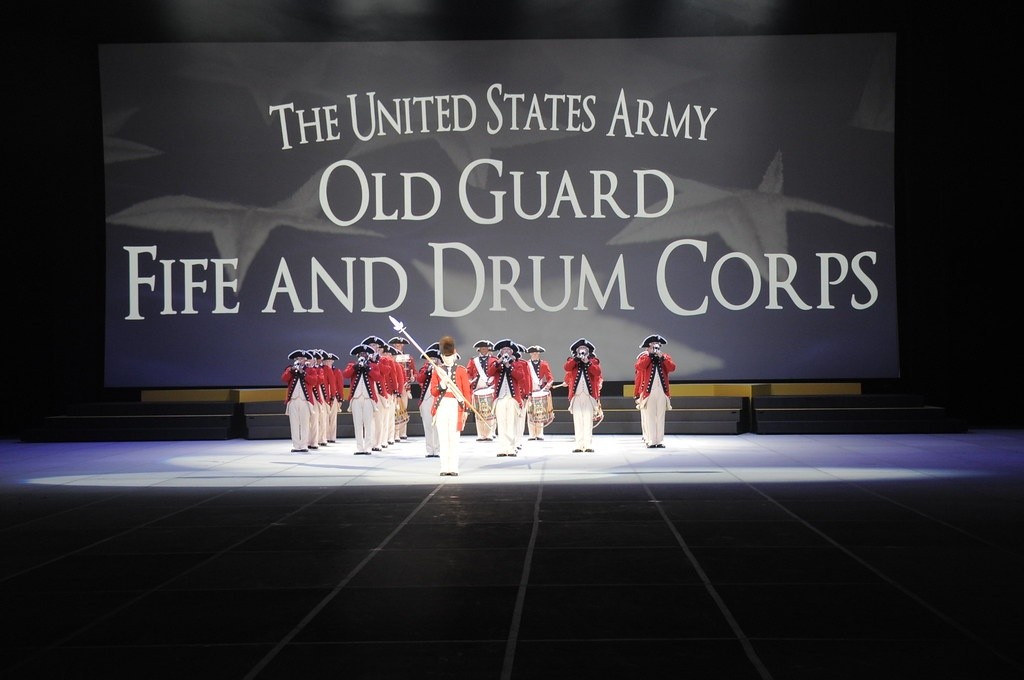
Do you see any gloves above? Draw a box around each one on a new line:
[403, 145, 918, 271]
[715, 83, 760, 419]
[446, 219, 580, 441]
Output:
[440, 376, 450, 390]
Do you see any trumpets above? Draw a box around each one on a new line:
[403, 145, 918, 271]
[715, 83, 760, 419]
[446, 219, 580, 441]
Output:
[653, 344, 660, 353]
[502, 353, 509, 360]
[578, 348, 587, 357]
[358, 356, 366, 363]
[294, 360, 301, 368]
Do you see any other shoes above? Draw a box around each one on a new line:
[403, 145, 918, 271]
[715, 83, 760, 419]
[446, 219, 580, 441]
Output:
[497, 445, 522, 457]
[440, 472, 458, 476]
[573, 449, 583, 452]
[291, 440, 335, 453]
[354, 436, 407, 455]
[642, 437, 665, 448]
[476, 438, 493, 441]
[426, 455, 439, 458]
[537, 437, 544, 440]
[528, 437, 536, 441]
[584, 448, 594, 452]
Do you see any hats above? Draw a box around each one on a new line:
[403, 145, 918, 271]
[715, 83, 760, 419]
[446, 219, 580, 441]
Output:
[288, 348, 340, 361]
[349, 336, 410, 355]
[474, 339, 545, 359]
[639, 335, 666, 348]
[420, 337, 461, 364]
[570, 338, 595, 359]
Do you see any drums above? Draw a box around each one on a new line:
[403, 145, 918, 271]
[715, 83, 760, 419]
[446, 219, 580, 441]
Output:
[473, 388, 496, 422]
[394, 396, 409, 425]
[593, 398, 604, 423]
[526, 389, 554, 423]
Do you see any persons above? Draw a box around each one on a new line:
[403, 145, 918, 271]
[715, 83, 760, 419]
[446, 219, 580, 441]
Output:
[564, 338, 604, 452]
[344, 335, 418, 455]
[417, 337, 554, 477]
[281, 349, 343, 452]
[634, 334, 676, 448]
